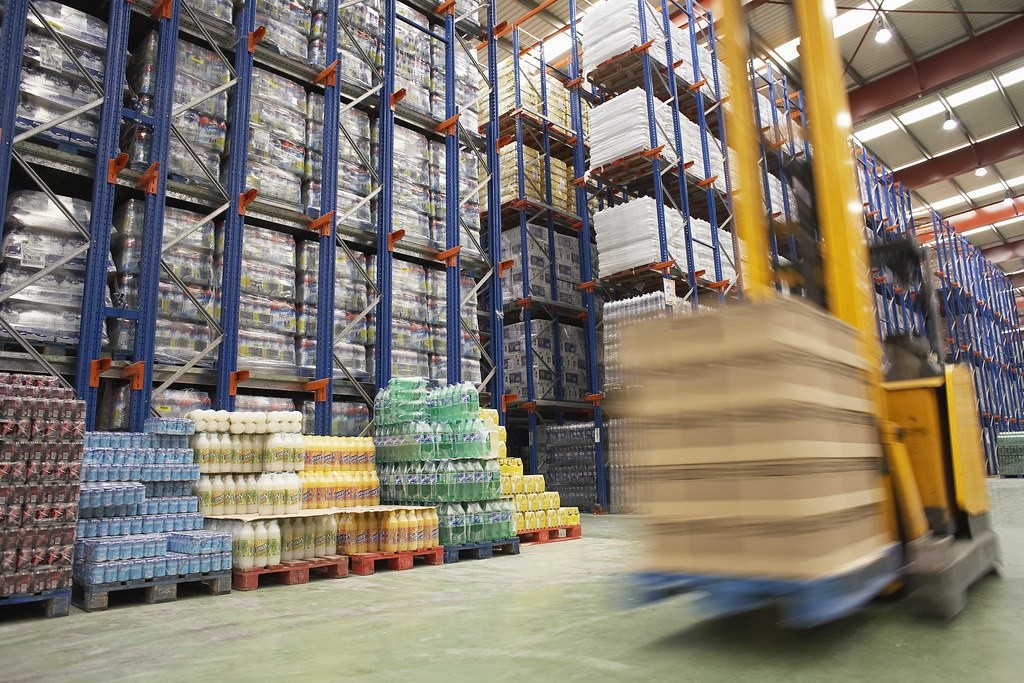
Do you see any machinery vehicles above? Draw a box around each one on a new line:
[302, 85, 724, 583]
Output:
[610, 0, 1008, 635]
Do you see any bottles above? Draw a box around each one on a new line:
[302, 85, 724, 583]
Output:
[996, 431, 1023, 478]
[3, 3, 493, 380]
[546, 290, 717, 512]
[184, 377, 518, 572]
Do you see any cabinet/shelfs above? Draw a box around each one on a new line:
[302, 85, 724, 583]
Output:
[0, 0, 1022, 512]
